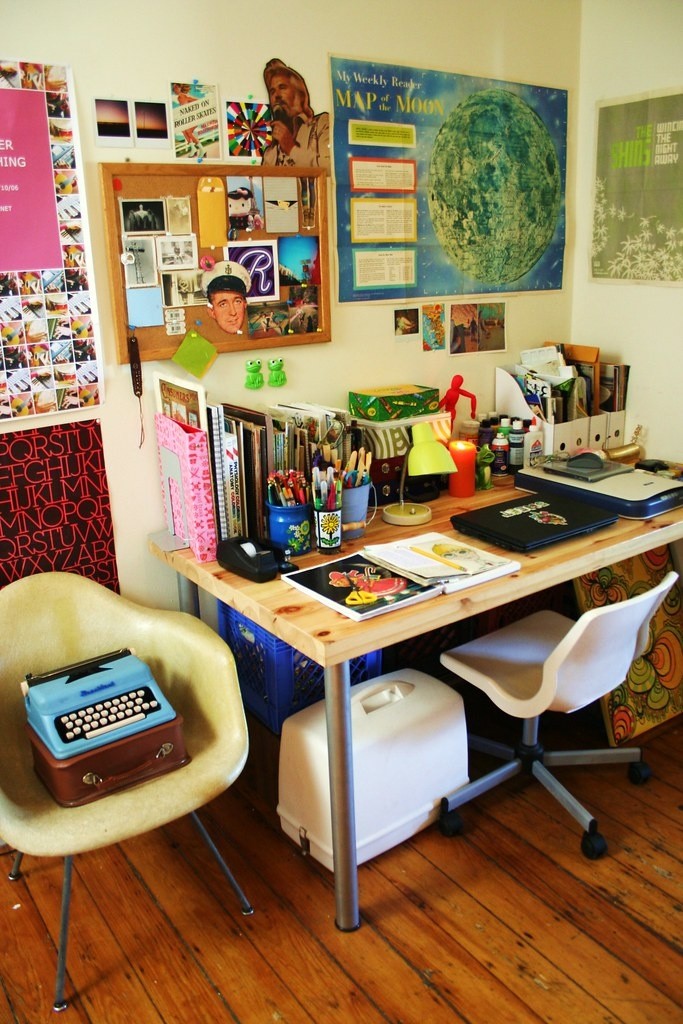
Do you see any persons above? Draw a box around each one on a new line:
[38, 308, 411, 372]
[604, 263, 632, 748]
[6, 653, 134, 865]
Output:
[200, 260, 251, 333]
[173, 243, 184, 264]
[261, 313, 267, 333]
[130, 204, 156, 232]
[171, 83, 207, 159]
[284, 286, 318, 335]
[263, 57, 331, 176]
[450, 310, 488, 354]
[439, 375, 477, 434]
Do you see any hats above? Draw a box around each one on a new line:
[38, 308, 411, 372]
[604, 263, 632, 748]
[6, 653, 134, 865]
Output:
[201, 261, 251, 298]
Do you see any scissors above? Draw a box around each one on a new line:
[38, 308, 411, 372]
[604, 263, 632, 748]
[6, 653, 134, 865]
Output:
[344, 572, 378, 605]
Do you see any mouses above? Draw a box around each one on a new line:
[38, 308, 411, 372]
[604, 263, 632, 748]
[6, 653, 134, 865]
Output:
[566, 452, 604, 469]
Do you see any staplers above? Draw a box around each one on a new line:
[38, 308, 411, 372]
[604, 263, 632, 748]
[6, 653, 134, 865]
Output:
[257, 537, 300, 573]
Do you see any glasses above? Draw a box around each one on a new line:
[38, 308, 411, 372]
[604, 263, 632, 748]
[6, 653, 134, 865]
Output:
[533, 452, 569, 468]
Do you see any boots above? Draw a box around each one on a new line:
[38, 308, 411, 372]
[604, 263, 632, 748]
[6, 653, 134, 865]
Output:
[187, 141, 198, 158]
[196, 141, 207, 159]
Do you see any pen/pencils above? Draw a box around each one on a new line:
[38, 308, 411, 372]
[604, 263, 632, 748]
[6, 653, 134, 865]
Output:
[410, 546, 468, 573]
[342, 447, 373, 489]
[310, 444, 343, 511]
[266, 467, 312, 507]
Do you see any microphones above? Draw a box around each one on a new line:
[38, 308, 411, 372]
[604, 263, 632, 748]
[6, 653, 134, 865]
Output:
[272, 105, 283, 144]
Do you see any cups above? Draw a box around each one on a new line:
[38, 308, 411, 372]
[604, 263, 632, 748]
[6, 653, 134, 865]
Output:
[309, 504, 342, 557]
[265, 499, 312, 558]
[339, 482, 372, 542]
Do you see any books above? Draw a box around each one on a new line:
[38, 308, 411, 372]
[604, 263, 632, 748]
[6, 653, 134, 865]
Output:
[511, 342, 630, 450]
[280, 531, 521, 622]
[154, 369, 366, 563]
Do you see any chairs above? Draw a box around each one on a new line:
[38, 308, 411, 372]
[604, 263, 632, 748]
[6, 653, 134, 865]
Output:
[0, 571, 254, 1012]
[437, 571, 679, 857]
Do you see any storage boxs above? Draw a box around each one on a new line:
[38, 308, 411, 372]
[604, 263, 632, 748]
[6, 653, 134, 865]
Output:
[348, 413, 452, 458]
[349, 384, 440, 422]
[216, 594, 383, 736]
[274, 668, 472, 877]
[371, 458, 440, 507]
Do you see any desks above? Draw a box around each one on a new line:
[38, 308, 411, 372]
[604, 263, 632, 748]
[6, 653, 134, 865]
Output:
[148, 461, 683, 932]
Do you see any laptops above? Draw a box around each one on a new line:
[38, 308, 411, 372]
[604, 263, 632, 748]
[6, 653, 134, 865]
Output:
[451, 492, 619, 553]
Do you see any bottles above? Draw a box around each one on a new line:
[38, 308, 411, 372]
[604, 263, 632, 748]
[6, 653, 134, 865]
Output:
[458, 411, 544, 477]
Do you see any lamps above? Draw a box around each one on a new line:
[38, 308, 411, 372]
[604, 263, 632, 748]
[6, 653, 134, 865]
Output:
[382, 422, 457, 525]
